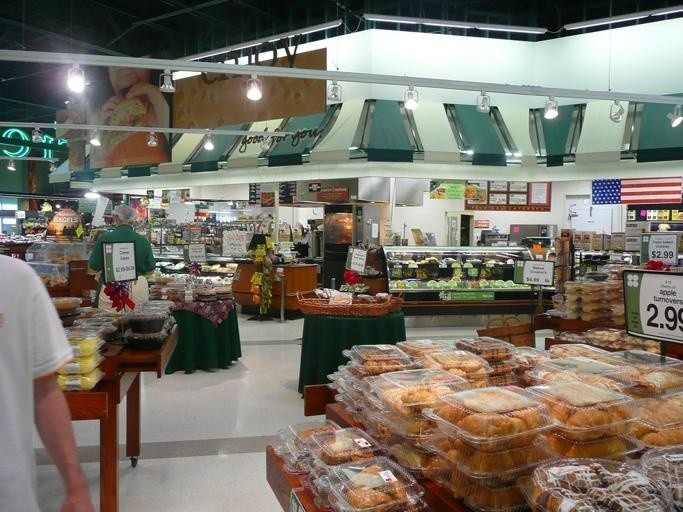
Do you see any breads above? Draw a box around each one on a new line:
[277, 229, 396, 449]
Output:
[551, 278, 626, 327]
[288, 337, 683, 512]
[562, 329, 661, 354]
[53, 298, 174, 388]
[149, 284, 234, 300]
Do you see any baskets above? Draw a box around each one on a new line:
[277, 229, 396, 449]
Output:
[295, 289, 403, 317]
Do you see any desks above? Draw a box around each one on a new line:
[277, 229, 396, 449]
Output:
[299, 311, 406, 397]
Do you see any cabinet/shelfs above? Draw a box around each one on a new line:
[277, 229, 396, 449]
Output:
[579, 252, 635, 284]
[265, 382, 461, 512]
[382, 246, 535, 298]
[536, 249, 574, 294]
[625, 205, 683, 264]
[533, 309, 683, 359]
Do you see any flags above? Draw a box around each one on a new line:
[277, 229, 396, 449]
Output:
[592, 177, 682, 205]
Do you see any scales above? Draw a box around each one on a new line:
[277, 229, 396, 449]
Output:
[519, 237, 551, 247]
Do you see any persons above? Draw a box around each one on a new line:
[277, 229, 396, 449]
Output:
[90, 66, 169, 168]
[89, 205, 156, 314]
[0, 254, 94, 512]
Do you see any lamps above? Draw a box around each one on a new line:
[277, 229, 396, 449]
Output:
[665, 111, 683, 129]
[246, 79, 263, 100]
[326, 83, 342, 105]
[159, 72, 175, 93]
[609, 101, 623, 125]
[543, 98, 558, 119]
[404, 89, 419, 111]
[67, 65, 86, 95]
[476, 94, 490, 114]
[6, 129, 275, 171]
